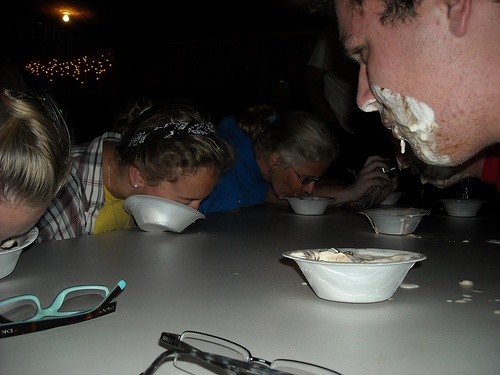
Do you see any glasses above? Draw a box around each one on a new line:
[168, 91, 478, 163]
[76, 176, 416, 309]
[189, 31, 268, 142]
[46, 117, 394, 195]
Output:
[286, 160, 320, 185]
[139, 330, 342, 375]
[0, 279, 127, 339]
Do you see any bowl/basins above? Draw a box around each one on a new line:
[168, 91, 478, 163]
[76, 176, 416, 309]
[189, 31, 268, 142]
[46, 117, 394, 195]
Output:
[356, 207, 430, 234]
[282, 248, 426, 303]
[280, 195, 336, 215]
[441, 199, 483, 217]
[123, 195, 205, 232]
[0, 227, 38, 279]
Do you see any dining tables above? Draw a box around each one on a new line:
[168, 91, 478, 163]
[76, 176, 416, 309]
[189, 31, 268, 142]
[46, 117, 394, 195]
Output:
[4, 199, 500, 372]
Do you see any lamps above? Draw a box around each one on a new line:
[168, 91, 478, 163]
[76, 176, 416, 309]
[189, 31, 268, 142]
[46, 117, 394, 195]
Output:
[60, 10, 72, 24]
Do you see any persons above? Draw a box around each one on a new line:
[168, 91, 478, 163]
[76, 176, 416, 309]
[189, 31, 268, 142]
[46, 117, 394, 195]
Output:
[337, 0, 500, 167]
[0, 86, 73, 243]
[200, 111, 500, 217]
[36, 98, 233, 245]
